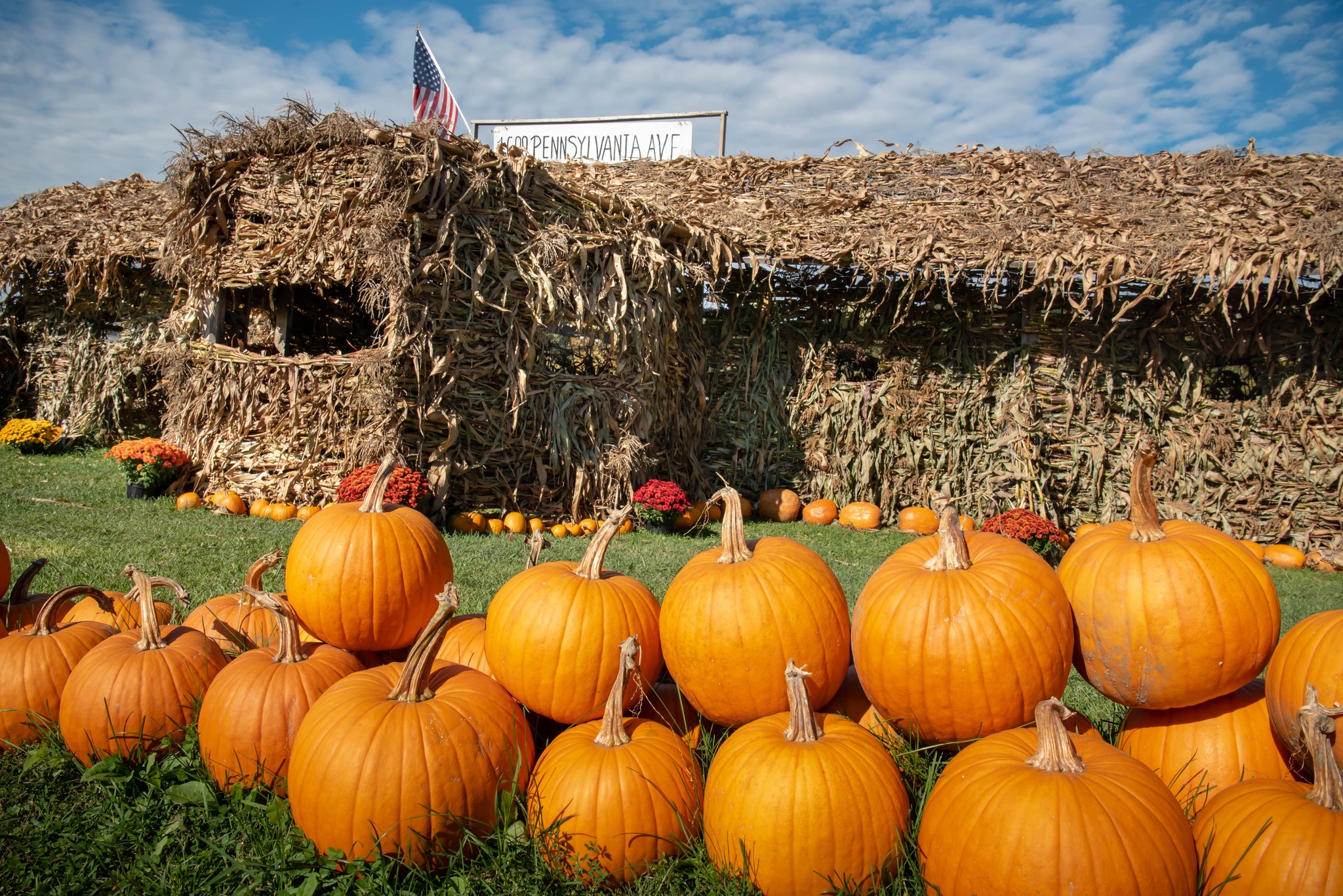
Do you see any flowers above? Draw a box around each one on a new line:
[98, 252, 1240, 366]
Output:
[336, 462, 433, 509]
[628, 479, 693, 523]
[104, 436, 189, 489]
[0, 418, 62, 447]
[980, 508, 1066, 560]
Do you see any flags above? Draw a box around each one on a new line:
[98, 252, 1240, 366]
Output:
[413, 28, 459, 143]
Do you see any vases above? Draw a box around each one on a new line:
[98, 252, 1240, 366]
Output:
[19, 446, 50, 457]
[126, 481, 160, 500]
[646, 520, 674, 535]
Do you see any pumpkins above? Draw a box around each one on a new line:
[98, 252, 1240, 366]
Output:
[0, 453, 1343, 896]
